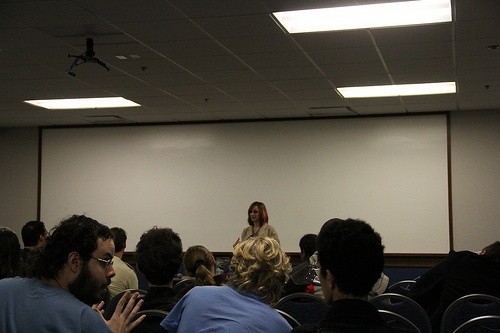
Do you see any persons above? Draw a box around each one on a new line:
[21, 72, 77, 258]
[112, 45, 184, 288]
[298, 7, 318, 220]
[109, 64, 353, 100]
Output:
[0, 214, 146, 333]
[160, 236, 294, 333]
[175, 245, 224, 294]
[232, 201, 281, 245]
[299, 234, 318, 262]
[288, 219, 411, 333]
[107, 227, 183, 319]
[475, 242, 500, 257]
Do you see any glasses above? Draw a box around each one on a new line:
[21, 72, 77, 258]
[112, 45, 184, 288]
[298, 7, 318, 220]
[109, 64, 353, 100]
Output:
[87, 255, 114, 269]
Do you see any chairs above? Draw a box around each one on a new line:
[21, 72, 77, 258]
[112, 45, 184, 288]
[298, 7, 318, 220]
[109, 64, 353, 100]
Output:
[276, 278, 500, 333]
[108, 286, 174, 333]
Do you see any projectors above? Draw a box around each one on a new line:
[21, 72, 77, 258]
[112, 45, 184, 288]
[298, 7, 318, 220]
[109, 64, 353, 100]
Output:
[66, 57, 109, 85]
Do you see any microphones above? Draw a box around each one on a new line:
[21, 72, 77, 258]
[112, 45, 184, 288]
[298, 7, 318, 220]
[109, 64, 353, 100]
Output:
[177, 273, 182, 279]
